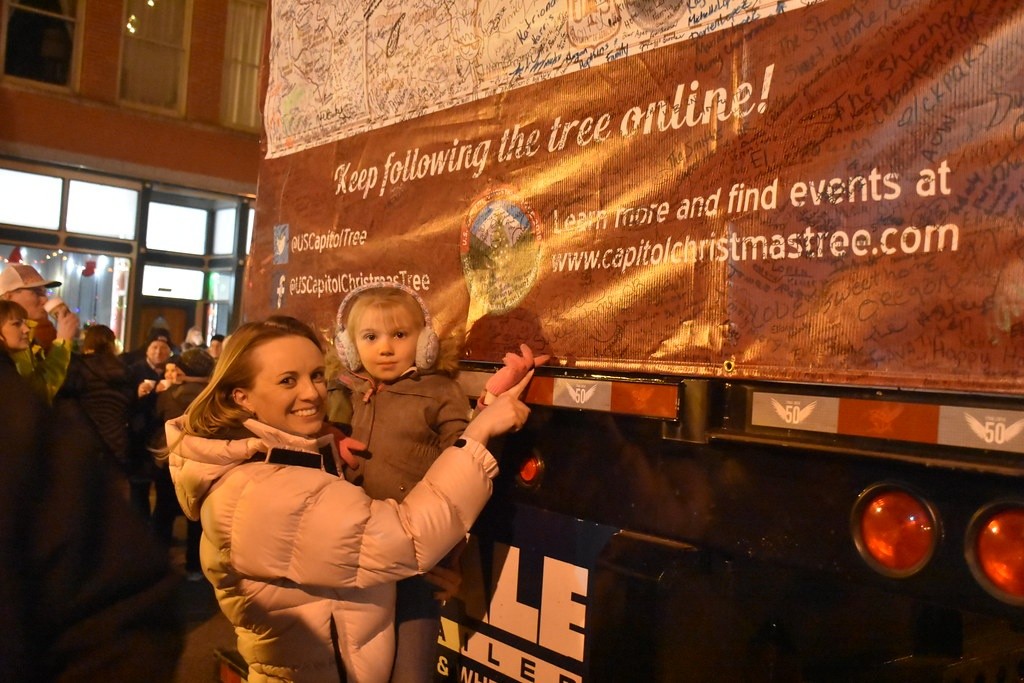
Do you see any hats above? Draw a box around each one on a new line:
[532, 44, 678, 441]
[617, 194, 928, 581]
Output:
[143, 327, 174, 353]
[0, 264, 62, 298]
[166, 356, 182, 364]
[210, 334, 224, 342]
[175, 349, 213, 377]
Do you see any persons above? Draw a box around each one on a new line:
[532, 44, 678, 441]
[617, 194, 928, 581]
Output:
[319, 280, 550, 683]
[147, 313, 534, 683]
[0, 261, 226, 621]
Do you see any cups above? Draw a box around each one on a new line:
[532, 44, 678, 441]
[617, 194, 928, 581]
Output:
[44, 298, 71, 323]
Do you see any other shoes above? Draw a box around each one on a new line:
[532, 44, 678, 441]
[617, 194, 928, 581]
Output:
[186, 571, 204, 581]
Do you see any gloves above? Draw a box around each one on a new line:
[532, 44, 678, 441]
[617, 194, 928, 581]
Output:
[319, 425, 367, 470]
[485, 344, 550, 398]
